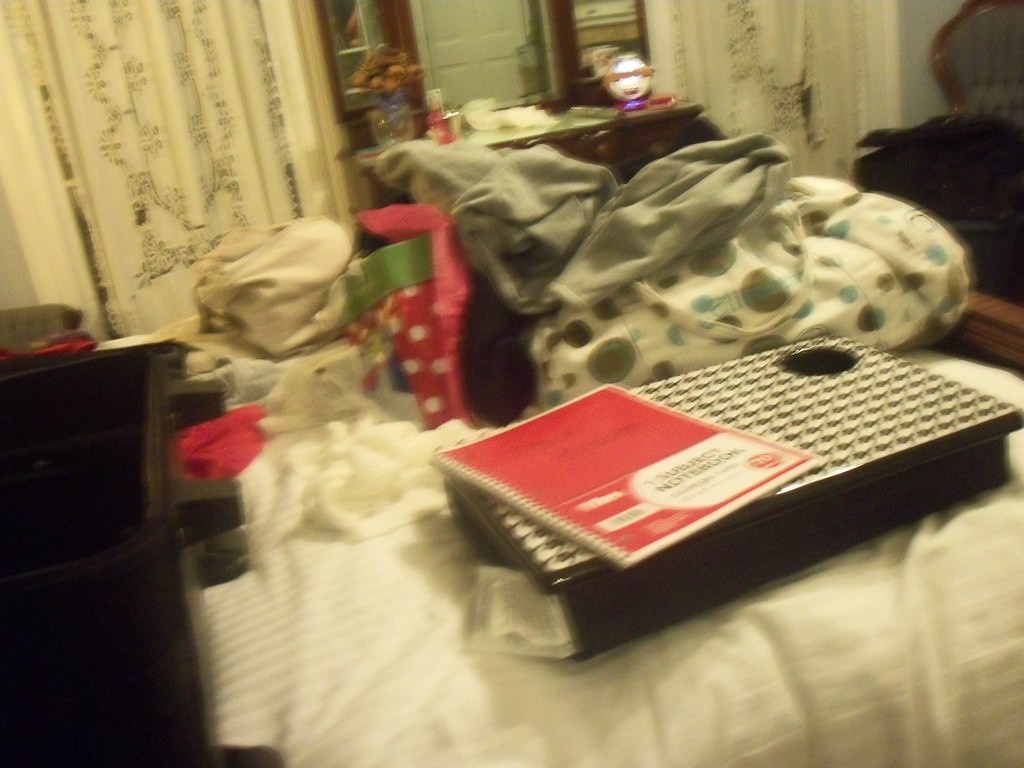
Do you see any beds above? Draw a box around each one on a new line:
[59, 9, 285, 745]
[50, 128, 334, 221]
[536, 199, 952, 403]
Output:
[93, 318, 1024, 768]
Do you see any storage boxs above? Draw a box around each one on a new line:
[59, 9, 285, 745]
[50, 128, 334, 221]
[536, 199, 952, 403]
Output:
[441, 336, 1023, 659]
[0, 348, 280, 768]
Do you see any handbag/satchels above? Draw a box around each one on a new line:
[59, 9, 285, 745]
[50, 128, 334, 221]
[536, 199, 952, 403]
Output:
[531, 176, 966, 409]
[194, 218, 353, 356]
[357, 204, 536, 430]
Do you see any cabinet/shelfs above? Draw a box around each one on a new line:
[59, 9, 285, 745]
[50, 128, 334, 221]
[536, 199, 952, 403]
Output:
[314, 0, 705, 185]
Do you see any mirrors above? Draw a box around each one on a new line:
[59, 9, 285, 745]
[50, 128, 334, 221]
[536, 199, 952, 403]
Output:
[402, 0, 573, 122]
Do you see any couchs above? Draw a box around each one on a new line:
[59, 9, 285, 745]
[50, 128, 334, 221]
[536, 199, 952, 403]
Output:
[851, 0, 1024, 365]
